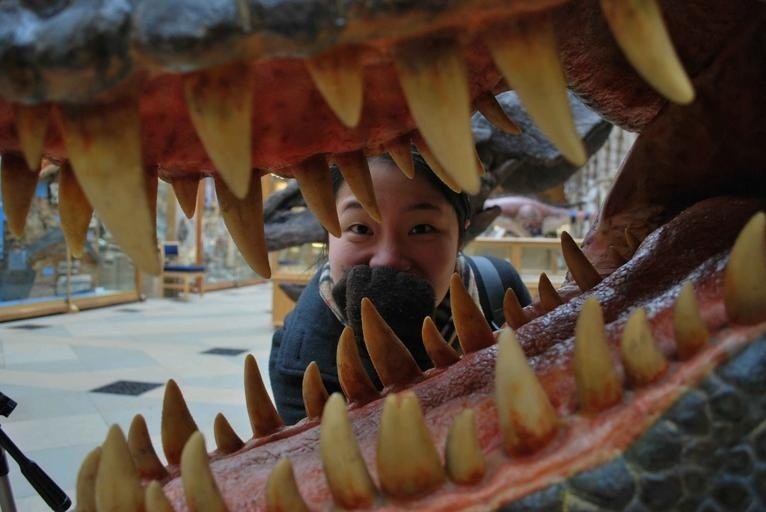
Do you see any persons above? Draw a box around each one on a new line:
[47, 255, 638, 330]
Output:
[269, 145, 531, 423]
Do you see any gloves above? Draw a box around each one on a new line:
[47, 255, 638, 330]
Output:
[329, 265, 437, 373]
